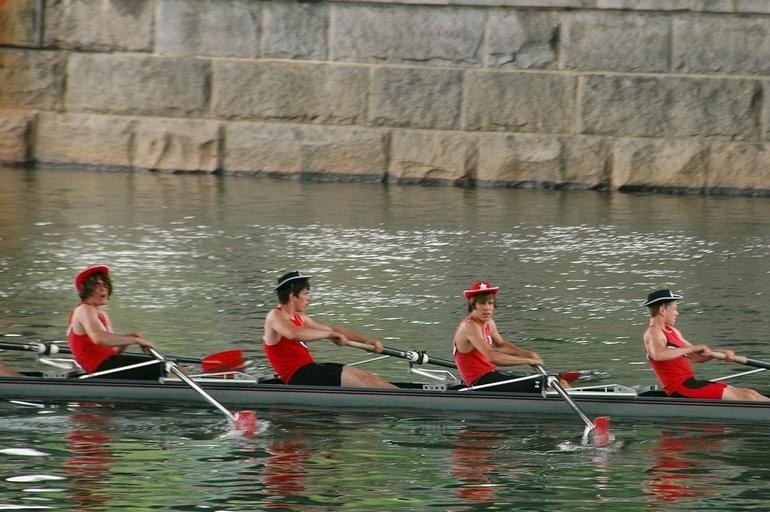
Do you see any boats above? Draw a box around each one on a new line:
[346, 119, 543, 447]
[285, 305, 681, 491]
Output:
[0, 364, 770, 431]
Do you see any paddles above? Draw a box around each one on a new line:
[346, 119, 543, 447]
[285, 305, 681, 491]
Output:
[148, 349, 256, 437]
[1, 343, 244, 374]
[535, 364, 608, 445]
[344, 342, 581, 384]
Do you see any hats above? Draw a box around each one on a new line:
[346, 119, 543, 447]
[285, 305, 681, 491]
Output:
[76, 265, 110, 289]
[274, 271, 312, 290]
[464, 282, 499, 299]
[640, 289, 683, 306]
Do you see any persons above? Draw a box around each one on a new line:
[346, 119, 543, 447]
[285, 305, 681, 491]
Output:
[62, 402, 115, 506]
[261, 268, 404, 390]
[642, 421, 719, 502]
[642, 287, 770, 403]
[449, 421, 505, 504]
[261, 421, 303, 510]
[65, 264, 187, 382]
[452, 280, 571, 394]
